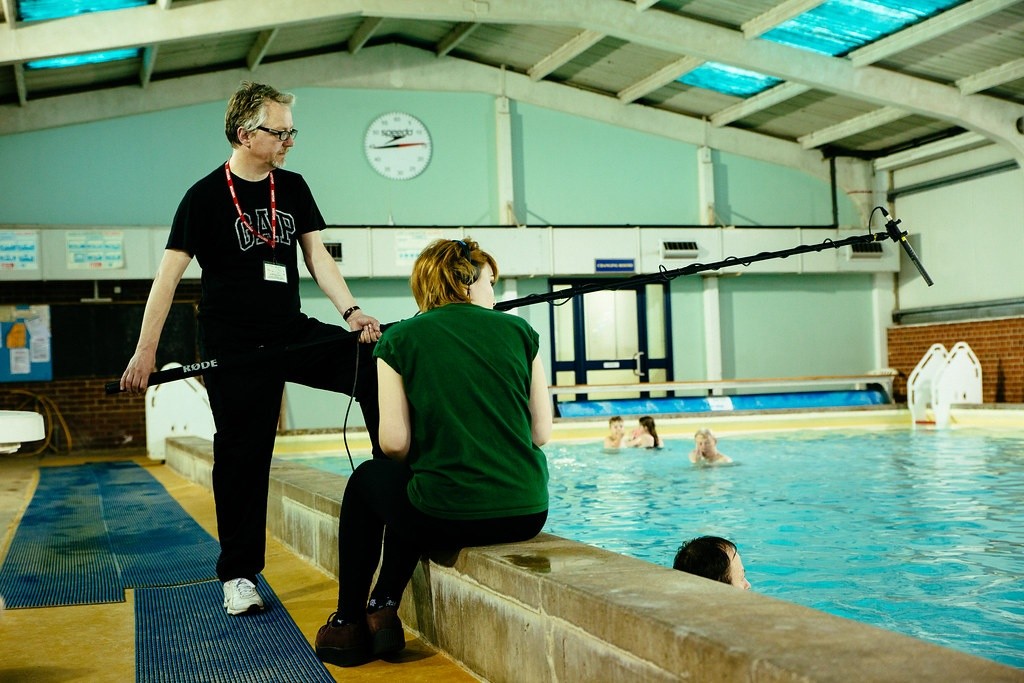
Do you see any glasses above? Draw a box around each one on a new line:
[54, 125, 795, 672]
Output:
[257, 126, 298, 141]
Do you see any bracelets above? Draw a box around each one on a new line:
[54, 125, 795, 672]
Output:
[339, 305, 360, 320]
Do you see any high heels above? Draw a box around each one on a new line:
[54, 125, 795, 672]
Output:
[316, 608, 406, 668]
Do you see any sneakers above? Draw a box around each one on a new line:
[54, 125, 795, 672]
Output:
[223, 577, 265, 615]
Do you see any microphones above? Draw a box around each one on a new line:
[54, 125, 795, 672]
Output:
[879, 207, 934, 287]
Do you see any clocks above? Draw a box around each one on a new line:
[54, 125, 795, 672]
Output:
[364, 112, 431, 180]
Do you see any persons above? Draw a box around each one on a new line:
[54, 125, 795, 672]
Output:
[689, 430, 734, 466]
[673, 535, 752, 598]
[314, 239, 554, 669]
[603, 414, 664, 452]
[119, 78, 390, 616]
